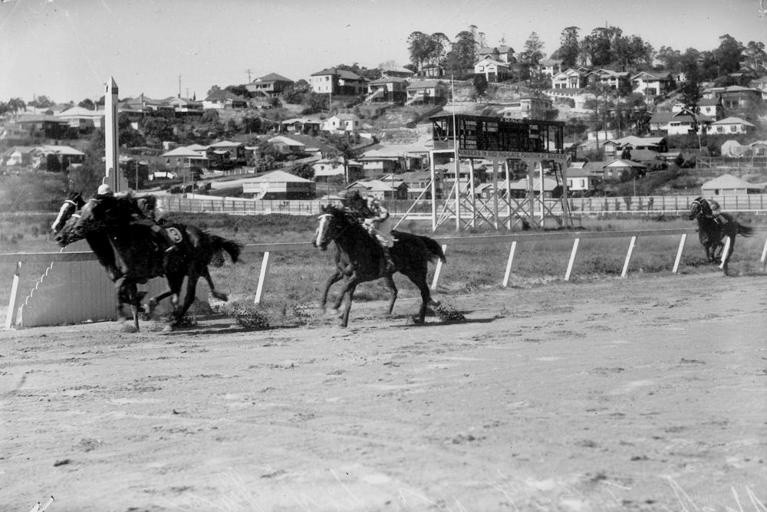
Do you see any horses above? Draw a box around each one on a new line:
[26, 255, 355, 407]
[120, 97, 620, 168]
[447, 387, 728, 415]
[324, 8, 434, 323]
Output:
[689, 197, 756, 262]
[50, 193, 87, 234]
[72, 195, 247, 332]
[55, 201, 223, 313]
[310, 206, 447, 329]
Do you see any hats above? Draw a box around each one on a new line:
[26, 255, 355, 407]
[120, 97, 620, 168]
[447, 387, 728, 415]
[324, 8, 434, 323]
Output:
[97, 183, 113, 196]
[340, 189, 360, 207]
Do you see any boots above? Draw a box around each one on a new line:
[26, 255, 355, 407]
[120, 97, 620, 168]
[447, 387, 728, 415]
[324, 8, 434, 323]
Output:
[382, 245, 395, 272]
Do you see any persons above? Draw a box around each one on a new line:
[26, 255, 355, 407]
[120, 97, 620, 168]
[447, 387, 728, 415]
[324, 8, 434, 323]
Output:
[342, 191, 396, 275]
[97, 185, 176, 256]
[707, 199, 729, 235]
